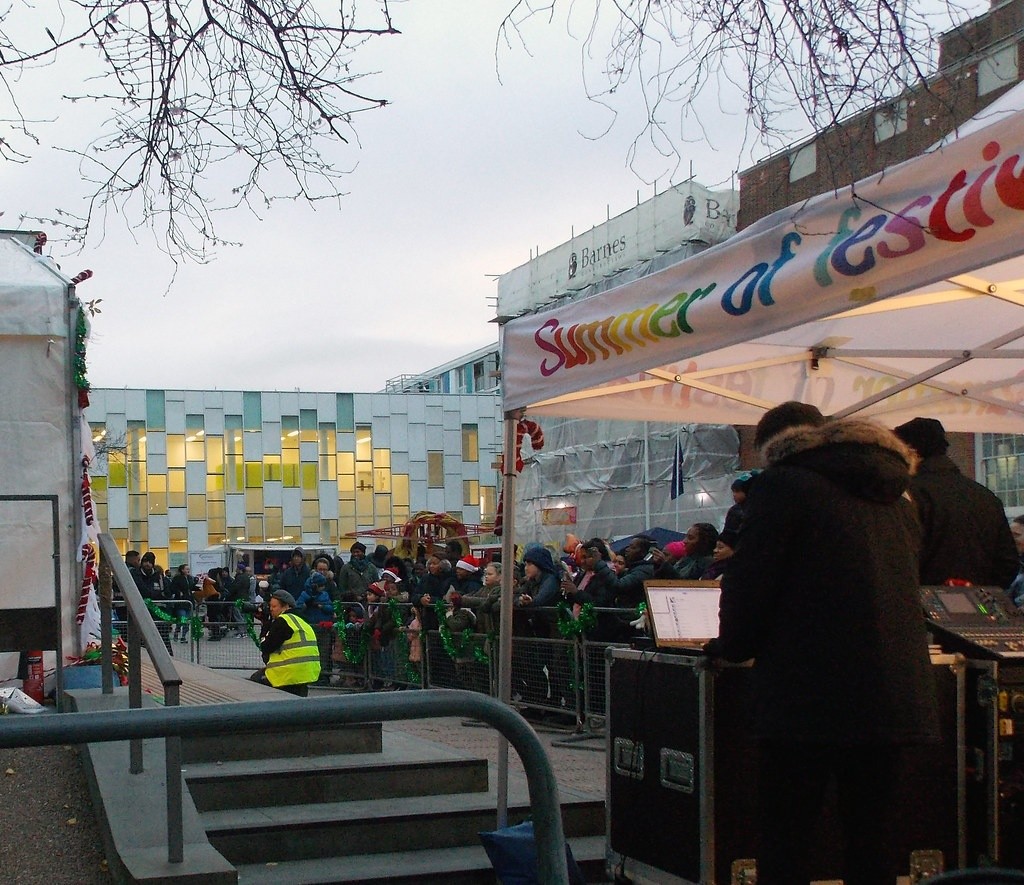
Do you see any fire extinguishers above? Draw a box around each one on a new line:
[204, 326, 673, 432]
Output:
[17, 624, 45, 708]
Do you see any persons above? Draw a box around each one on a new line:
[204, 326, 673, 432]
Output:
[723, 469, 759, 533]
[112, 541, 616, 692]
[698, 530, 737, 580]
[588, 534, 655, 643]
[703, 401, 946, 885]
[247, 589, 321, 697]
[650, 523, 719, 580]
[1010, 514, 1024, 555]
[894, 417, 1020, 590]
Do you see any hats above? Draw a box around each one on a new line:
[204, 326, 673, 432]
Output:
[271, 589, 297, 608]
[894, 418, 949, 454]
[223, 567, 229, 574]
[142, 552, 155, 562]
[237, 564, 245, 571]
[523, 547, 552, 571]
[381, 567, 399, 579]
[456, 554, 479, 573]
[368, 581, 386, 596]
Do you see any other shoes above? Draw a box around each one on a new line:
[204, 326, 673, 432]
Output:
[206, 635, 221, 641]
[180, 640, 188, 643]
[232, 633, 248, 639]
[174, 638, 178, 641]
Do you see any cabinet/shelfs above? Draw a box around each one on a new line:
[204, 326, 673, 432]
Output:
[605, 647, 965, 884]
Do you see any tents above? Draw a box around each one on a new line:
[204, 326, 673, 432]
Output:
[498, 77, 1024, 829]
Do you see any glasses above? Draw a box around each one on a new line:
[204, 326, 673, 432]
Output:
[142, 558, 154, 564]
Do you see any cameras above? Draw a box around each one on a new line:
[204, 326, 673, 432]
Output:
[643, 547, 659, 562]
[561, 570, 573, 585]
[238, 599, 269, 620]
[587, 546, 599, 558]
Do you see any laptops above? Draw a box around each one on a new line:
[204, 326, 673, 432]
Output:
[643, 579, 740, 655]
